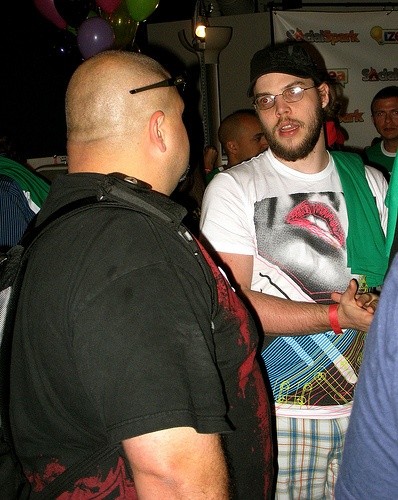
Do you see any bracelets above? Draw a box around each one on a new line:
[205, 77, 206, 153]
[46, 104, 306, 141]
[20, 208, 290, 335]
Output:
[328, 304, 344, 335]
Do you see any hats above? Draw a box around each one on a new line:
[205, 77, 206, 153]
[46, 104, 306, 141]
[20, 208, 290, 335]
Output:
[247, 36, 330, 98]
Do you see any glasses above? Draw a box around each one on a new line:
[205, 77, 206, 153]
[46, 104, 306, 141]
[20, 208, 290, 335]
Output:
[130, 73, 188, 95]
[251, 84, 313, 112]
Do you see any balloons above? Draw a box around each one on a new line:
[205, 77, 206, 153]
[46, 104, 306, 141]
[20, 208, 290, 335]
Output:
[36, 0, 159, 59]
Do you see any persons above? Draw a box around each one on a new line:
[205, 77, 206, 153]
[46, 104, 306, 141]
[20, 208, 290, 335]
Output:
[0, 39, 398, 500]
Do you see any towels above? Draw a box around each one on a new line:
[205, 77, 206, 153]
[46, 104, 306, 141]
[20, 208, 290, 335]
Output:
[385, 149, 398, 258]
[206, 169, 218, 184]
[331, 151, 389, 287]
[0, 156, 51, 208]
